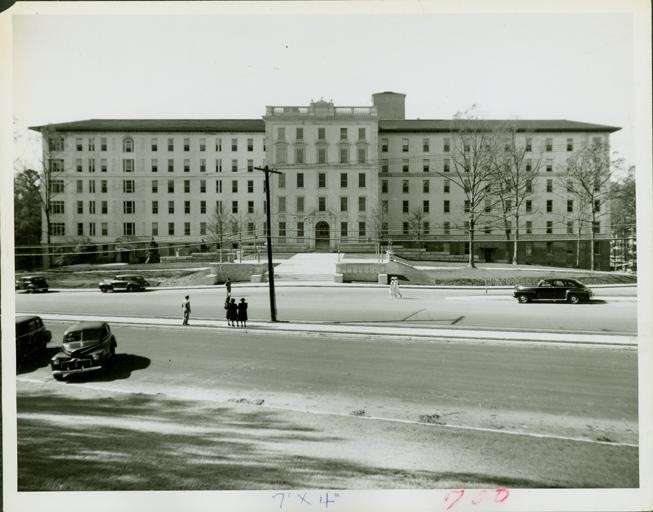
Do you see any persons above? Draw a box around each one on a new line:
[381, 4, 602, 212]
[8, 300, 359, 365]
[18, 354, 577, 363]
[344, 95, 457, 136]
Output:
[226, 297, 239, 327]
[224, 276, 231, 296]
[181, 294, 191, 325]
[394, 277, 402, 298]
[388, 277, 397, 299]
[236, 297, 248, 327]
[224, 296, 231, 326]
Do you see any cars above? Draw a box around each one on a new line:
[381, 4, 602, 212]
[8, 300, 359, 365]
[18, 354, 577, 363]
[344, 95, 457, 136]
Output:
[51, 321, 117, 383]
[512, 277, 594, 304]
[15, 274, 49, 296]
[97, 273, 150, 294]
[16, 315, 52, 365]
